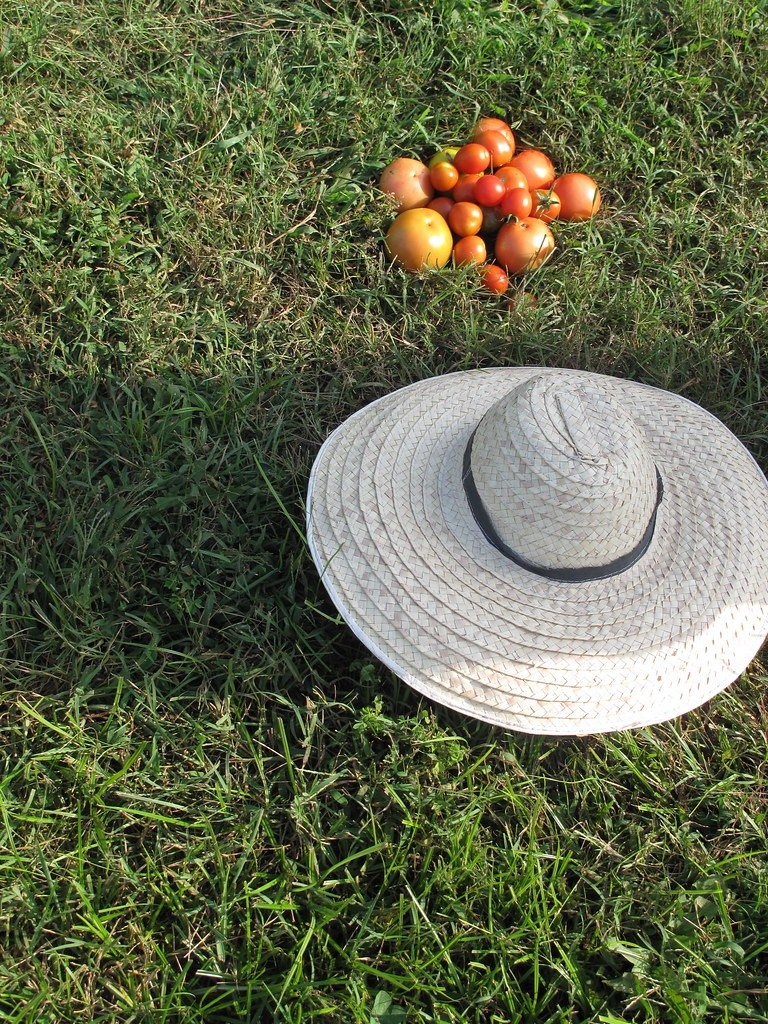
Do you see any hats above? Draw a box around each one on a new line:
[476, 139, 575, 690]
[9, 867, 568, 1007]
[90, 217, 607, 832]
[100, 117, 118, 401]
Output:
[304, 365, 767, 736]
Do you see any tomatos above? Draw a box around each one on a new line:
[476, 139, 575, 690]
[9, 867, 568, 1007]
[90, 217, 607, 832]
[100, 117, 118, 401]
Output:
[380, 117, 600, 311]
